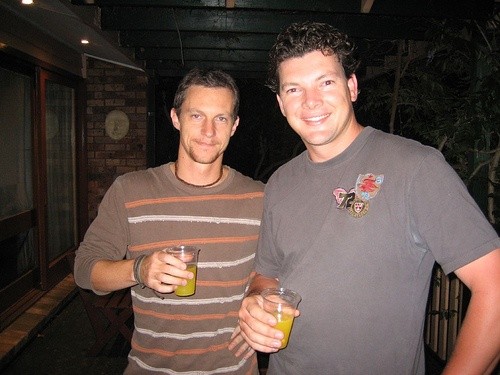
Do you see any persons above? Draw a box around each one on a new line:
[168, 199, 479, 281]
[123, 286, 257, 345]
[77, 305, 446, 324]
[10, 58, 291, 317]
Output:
[239, 25, 500, 375]
[74, 65, 267, 375]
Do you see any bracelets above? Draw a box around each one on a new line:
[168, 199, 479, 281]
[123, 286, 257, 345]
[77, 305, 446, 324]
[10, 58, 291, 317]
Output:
[133, 255, 146, 289]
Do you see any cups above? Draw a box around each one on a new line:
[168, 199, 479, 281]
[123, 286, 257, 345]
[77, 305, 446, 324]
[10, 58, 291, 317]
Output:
[261, 288, 302, 349]
[166, 246, 201, 297]
